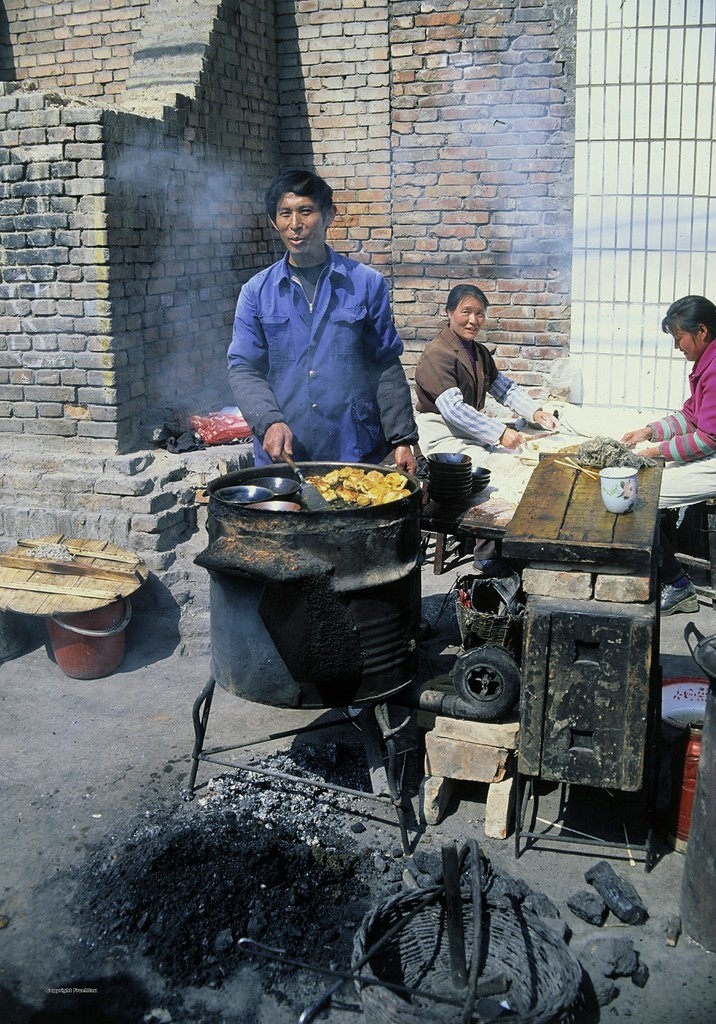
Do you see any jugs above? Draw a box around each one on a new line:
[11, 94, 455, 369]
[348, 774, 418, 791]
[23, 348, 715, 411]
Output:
[666, 719, 704, 854]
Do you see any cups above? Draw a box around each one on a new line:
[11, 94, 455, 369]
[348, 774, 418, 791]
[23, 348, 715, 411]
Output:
[600, 468, 638, 514]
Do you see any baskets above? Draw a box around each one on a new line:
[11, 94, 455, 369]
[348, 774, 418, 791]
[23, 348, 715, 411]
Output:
[352, 839, 582, 1024]
[453, 574, 526, 660]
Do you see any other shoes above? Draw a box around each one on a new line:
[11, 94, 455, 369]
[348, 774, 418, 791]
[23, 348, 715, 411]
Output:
[473, 557, 517, 579]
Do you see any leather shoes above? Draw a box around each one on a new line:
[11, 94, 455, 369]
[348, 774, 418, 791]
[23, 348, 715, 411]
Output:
[660, 580, 699, 615]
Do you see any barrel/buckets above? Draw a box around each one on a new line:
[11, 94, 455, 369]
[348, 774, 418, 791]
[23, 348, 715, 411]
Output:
[44, 598, 132, 678]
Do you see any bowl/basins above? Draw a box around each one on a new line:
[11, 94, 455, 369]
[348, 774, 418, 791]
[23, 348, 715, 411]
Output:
[214, 487, 274, 504]
[426, 453, 490, 506]
[244, 501, 301, 511]
[242, 478, 300, 500]
[654, 676, 709, 741]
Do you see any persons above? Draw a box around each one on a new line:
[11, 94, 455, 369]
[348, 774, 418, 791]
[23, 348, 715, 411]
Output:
[227, 172, 420, 479]
[618, 294, 716, 614]
[414, 281, 554, 578]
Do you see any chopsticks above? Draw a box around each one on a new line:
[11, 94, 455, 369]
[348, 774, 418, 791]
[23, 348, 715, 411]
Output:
[553, 457, 599, 480]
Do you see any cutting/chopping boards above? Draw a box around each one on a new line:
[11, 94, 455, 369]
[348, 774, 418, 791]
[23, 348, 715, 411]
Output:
[501, 453, 664, 564]
[420, 428, 594, 540]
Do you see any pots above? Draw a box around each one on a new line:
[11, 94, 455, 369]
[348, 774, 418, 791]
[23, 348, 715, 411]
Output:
[684, 622, 716, 688]
[207, 461, 419, 512]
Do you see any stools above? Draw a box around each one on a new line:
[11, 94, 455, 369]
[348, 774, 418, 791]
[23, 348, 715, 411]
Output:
[670, 498, 716, 610]
[417, 471, 464, 575]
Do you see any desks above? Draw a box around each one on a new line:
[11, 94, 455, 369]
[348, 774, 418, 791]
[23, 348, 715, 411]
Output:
[501, 453, 666, 871]
